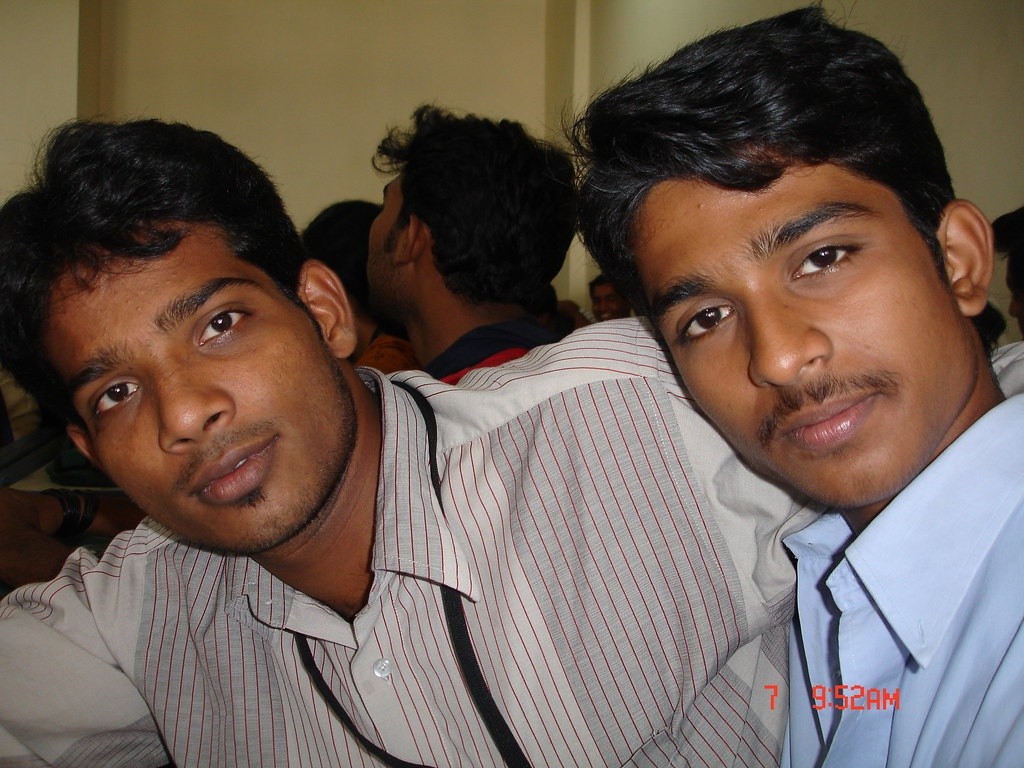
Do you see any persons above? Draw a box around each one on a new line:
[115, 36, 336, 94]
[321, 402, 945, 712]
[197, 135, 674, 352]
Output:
[0, 486, 148, 591]
[300, 106, 632, 386]
[991, 204, 1024, 344]
[0, 0, 1024, 768]
[545, 0, 1024, 768]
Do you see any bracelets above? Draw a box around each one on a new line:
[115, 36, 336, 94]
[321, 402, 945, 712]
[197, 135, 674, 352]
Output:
[40, 487, 101, 538]
